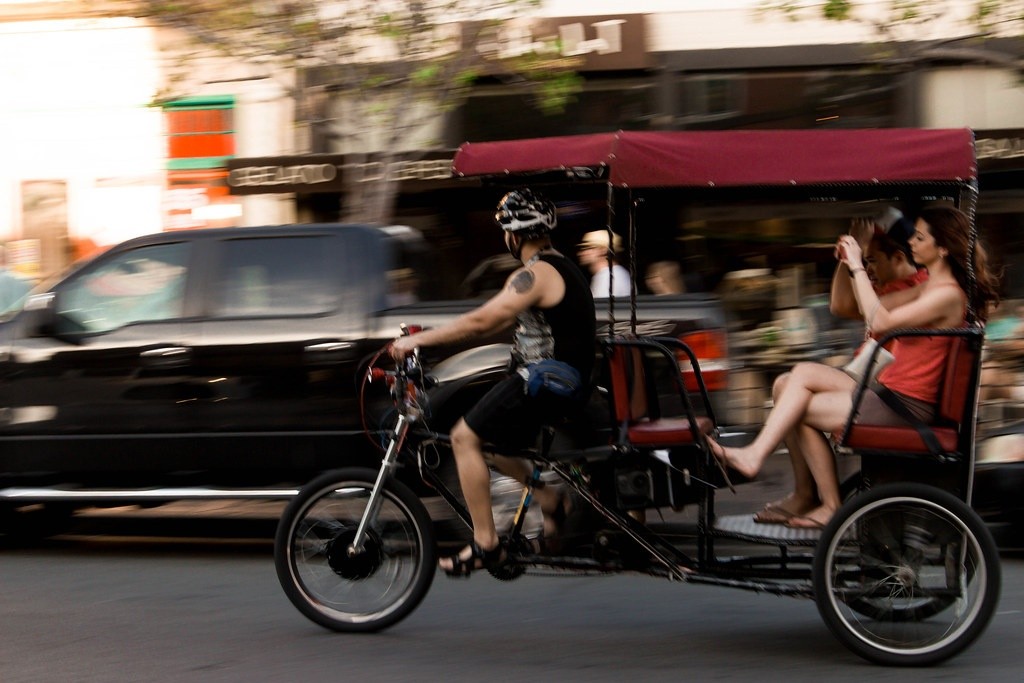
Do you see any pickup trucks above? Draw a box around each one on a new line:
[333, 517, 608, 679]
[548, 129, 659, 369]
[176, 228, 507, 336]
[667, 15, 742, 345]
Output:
[0, 219, 733, 500]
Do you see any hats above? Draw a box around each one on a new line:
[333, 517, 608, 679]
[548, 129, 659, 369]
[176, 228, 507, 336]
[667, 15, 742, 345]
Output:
[577, 229, 622, 252]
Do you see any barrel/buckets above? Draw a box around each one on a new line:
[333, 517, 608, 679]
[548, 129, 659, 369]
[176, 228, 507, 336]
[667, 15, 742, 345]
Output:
[717, 367, 771, 425]
[675, 328, 726, 389]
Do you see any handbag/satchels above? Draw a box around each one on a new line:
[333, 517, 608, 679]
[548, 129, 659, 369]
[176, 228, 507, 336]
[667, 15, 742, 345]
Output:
[527, 359, 580, 414]
[844, 338, 894, 387]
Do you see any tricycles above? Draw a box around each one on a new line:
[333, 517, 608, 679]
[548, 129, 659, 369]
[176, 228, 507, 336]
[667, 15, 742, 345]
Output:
[264, 122, 1006, 670]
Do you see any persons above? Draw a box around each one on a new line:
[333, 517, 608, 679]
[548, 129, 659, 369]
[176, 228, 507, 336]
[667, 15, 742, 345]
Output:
[390, 187, 599, 574]
[581, 199, 968, 530]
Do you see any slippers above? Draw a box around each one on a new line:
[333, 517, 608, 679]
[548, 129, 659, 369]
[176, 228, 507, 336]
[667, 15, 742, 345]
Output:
[703, 432, 737, 495]
[783, 515, 826, 529]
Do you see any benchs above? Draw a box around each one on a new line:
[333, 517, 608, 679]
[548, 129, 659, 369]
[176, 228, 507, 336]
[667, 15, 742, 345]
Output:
[598, 348, 711, 447]
[833, 319, 977, 454]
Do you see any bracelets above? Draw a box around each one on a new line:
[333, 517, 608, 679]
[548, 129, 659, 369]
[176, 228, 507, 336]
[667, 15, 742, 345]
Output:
[852, 268, 866, 278]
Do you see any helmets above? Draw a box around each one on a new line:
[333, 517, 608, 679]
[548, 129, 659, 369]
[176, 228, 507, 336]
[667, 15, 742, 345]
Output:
[495, 188, 557, 232]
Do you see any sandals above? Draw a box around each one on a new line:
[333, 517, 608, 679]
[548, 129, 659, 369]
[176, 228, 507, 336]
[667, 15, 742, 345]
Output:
[753, 505, 799, 524]
[528, 530, 562, 555]
[445, 536, 511, 573]
